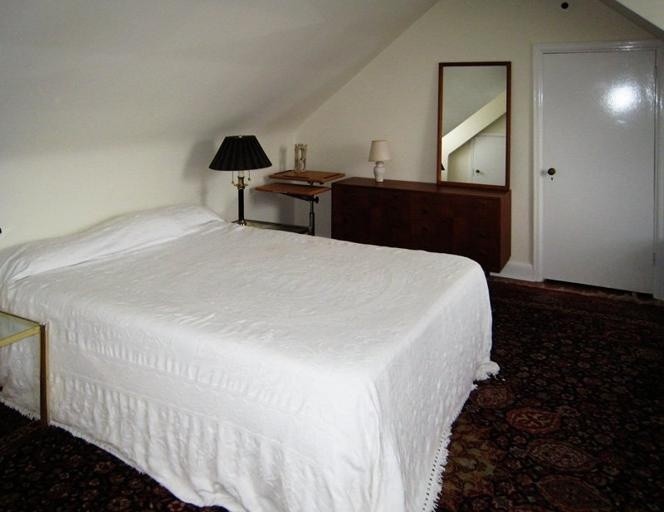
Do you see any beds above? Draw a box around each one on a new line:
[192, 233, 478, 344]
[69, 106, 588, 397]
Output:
[0, 200, 493, 510]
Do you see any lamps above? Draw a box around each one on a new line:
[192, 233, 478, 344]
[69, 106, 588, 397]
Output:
[209, 132, 272, 225]
[369, 138, 392, 184]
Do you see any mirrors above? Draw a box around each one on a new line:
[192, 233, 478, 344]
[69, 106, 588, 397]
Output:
[437, 60, 510, 190]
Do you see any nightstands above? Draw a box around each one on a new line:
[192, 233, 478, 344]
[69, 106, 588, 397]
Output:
[231, 216, 313, 234]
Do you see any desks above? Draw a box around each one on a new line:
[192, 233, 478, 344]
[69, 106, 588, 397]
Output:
[254, 169, 345, 236]
[0, 309, 50, 442]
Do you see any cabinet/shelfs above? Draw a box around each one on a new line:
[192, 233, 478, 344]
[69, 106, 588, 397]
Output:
[330, 173, 513, 273]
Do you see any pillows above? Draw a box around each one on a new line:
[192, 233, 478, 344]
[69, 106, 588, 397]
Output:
[3, 203, 216, 281]
